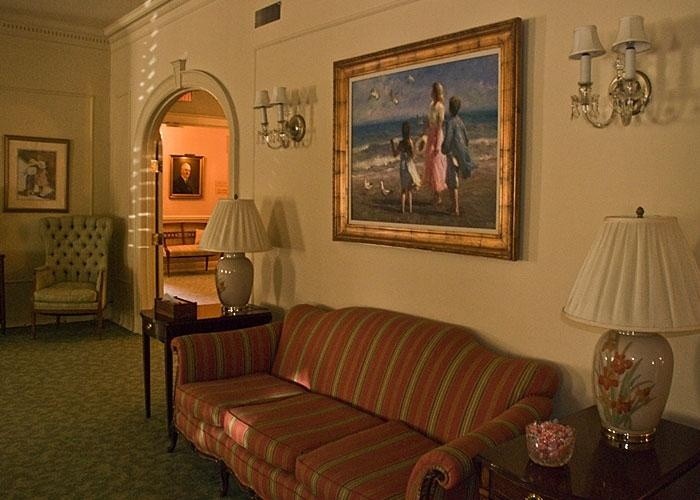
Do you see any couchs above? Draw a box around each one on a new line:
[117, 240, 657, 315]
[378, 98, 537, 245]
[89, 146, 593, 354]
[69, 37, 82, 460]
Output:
[168, 305, 562, 500]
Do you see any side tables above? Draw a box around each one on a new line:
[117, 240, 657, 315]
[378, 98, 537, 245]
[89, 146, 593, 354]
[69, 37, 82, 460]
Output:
[473, 403, 699, 499]
[138, 305, 273, 432]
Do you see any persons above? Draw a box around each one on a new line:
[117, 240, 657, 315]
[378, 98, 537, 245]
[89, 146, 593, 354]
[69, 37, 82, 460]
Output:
[442, 95, 475, 218]
[28, 155, 54, 197]
[422, 82, 449, 205]
[178, 162, 195, 194]
[390, 122, 421, 214]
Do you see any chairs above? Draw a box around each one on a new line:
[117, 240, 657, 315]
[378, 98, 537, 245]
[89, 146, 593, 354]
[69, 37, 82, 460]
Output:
[29, 215, 116, 338]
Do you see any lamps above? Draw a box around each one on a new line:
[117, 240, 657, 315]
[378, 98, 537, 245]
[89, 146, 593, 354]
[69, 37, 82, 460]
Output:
[562, 203, 699, 452]
[565, 13, 653, 128]
[199, 194, 274, 313]
[253, 85, 305, 150]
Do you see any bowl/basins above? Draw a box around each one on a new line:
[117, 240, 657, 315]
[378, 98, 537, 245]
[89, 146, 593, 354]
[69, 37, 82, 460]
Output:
[525, 426, 575, 466]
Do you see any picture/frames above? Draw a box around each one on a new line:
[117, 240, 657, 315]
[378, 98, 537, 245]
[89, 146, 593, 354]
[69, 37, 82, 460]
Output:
[168, 153, 206, 199]
[2, 134, 72, 213]
[329, 12, 524, 262]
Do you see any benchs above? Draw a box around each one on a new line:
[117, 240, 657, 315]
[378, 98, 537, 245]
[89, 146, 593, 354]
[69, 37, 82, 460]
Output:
[160, 231, 222, 277]
